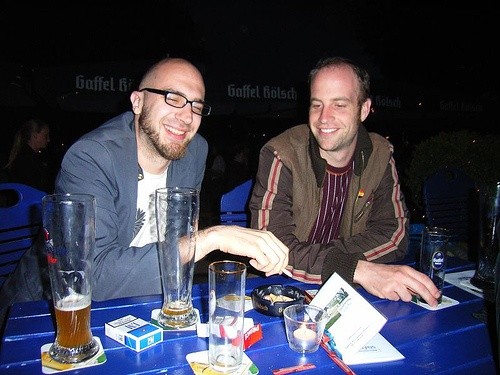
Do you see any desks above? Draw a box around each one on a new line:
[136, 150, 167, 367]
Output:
[0, 257, 497, 375]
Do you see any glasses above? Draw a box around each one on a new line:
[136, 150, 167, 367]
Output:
[138, 88, 212, 116]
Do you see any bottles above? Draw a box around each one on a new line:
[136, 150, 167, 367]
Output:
[470, 180, 500, 290]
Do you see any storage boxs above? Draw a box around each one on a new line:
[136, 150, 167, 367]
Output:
[196, 316, 264, 350]
[105, 315, 163, 353]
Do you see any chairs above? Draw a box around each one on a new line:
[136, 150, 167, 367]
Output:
[0, 184, 49, 288]
[219, 179, 426, 274]
[423, 170, 479, 244]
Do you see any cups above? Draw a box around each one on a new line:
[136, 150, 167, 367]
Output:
[208, 260, 247, 373]
[417, 225, 450, 304]
[283, 304, 329, 354]
[42, 192, 101, 365]
[154, 185, 200, 329]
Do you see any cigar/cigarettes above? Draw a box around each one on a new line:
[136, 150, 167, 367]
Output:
[267, 293, 292, 302]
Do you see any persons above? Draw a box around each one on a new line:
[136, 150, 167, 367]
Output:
[0, 57, 290, 325]
[249, 55, 440, 306]
[227, 147, 249, 187]
[9, 119, 56, 205]
